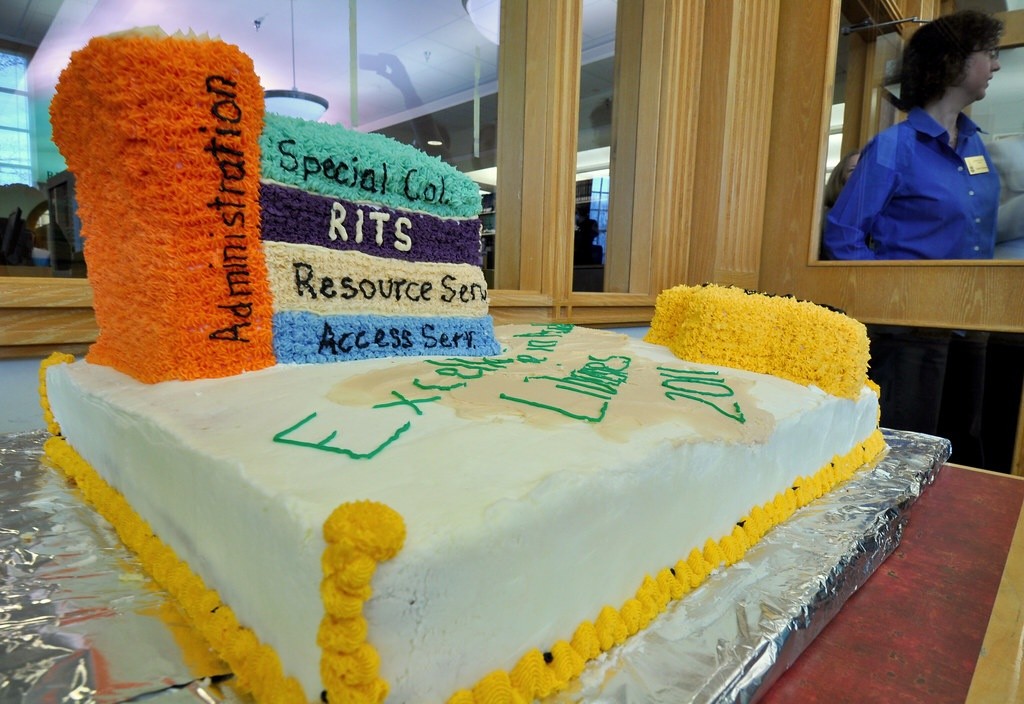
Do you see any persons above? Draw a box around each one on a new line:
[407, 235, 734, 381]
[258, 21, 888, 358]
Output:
[573, 203, 604, 291]
[982, 133, 1024, 473]
[818, 10, 1007, 465]
[34, 222, 73, 277]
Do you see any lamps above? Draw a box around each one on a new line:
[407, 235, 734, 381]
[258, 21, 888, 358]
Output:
[261, 2, 329, 120]
[461, 0, 501, 46]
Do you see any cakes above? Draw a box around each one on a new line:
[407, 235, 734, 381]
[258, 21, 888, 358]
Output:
[39, 22, 890, 704]
